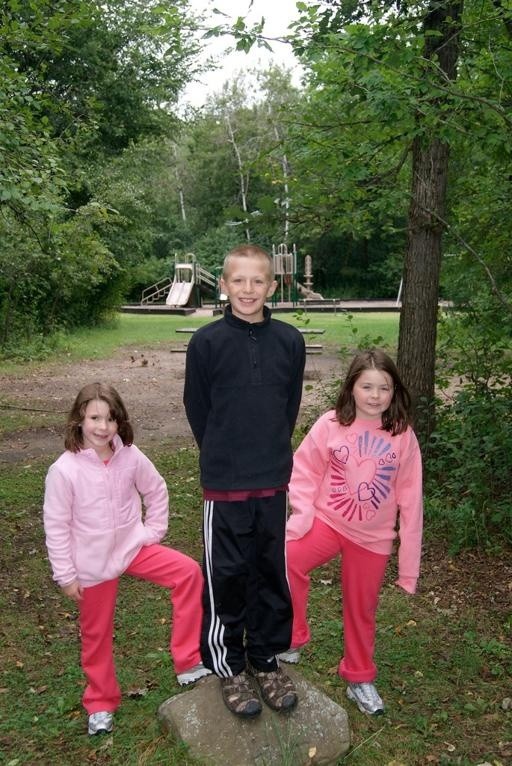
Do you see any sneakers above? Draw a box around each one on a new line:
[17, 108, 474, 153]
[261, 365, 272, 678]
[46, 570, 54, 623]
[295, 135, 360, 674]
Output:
[175, 662, 215, 686]
[278, 646, 302, 666]
[86, 711, 117, 735]
[345, 680, 386, 717]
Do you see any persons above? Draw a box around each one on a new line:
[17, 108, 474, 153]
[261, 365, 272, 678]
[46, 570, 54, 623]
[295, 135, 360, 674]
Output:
[273, 350, 424, 716]
[43, 381, 215, 736]
[182, 245, 305, 720]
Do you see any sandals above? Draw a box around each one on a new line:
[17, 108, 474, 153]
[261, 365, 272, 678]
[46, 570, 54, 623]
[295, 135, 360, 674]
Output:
[221, 672, 264, 719]
[252, 669, 298, 713]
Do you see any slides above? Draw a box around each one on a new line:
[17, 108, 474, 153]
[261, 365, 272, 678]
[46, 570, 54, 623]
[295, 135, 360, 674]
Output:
[167, 279, 194, 306]
